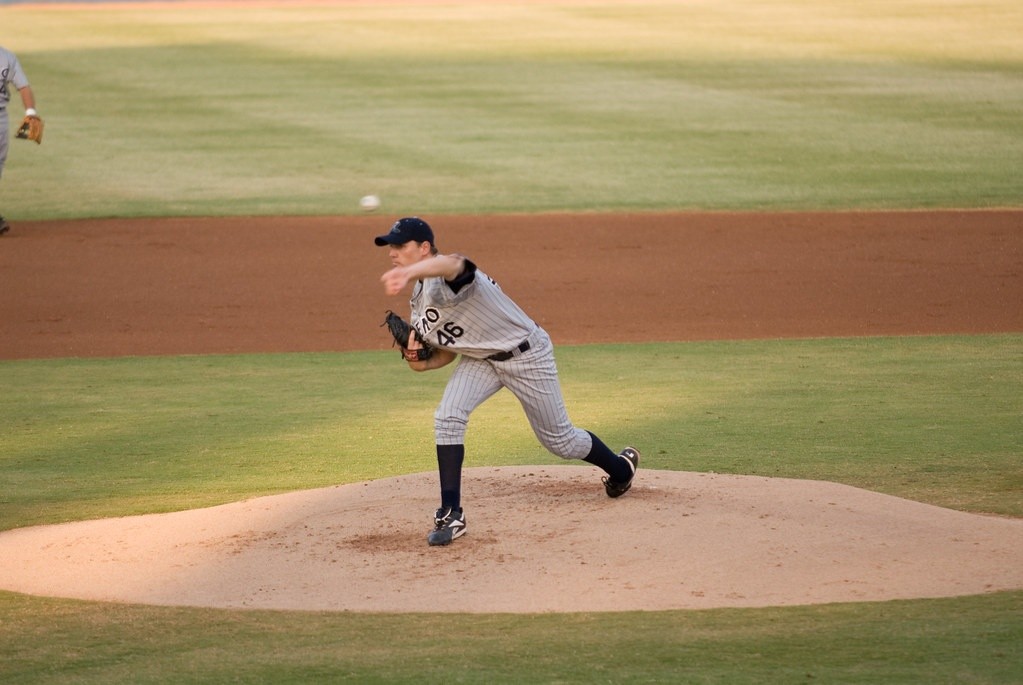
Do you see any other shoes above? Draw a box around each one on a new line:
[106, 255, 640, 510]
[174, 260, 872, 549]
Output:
[0, 215, 9, 234]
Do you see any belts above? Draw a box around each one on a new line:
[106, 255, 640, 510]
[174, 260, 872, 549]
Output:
[488, 322, 539, 361]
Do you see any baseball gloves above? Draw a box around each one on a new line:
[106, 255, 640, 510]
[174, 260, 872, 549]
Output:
[385, 311, 433, 361]
[15, 115, 43, 144]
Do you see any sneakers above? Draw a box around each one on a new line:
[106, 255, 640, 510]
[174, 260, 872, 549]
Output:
[428, 506, 467, 546]
[601, 446, 640, 498]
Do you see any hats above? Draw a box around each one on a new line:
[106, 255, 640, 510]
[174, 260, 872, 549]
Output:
[375, 218, 434, 247]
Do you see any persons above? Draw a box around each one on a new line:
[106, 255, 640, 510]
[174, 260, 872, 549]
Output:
[373, 217, 639, 547]
[0, 47, 44, 234]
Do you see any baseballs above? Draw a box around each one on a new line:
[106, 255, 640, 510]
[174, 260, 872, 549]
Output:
[361, 196, 378, 212]
